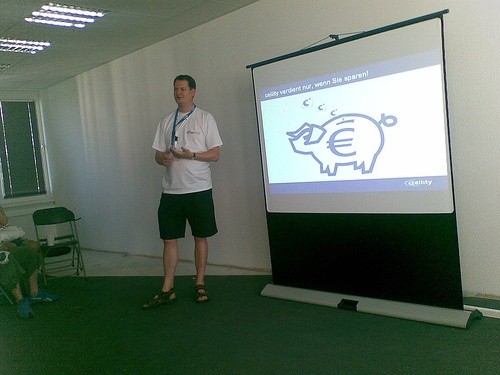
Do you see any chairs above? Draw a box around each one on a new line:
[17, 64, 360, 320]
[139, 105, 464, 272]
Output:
[32, 207, 89, 287]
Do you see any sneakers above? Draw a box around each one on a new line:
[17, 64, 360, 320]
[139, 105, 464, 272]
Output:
[15, 298, 34, 320]
[28, 292, 57, 304]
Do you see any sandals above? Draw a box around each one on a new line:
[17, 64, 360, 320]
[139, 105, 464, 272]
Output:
[142, 288, 178, 310]
[193, 284, 209, 303]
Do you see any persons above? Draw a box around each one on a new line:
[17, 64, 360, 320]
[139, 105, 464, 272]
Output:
[141, 75, 224, 309]
[0, 206, 60, 319]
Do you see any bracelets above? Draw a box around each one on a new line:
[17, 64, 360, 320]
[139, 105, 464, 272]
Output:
[192, 152, 195, 160]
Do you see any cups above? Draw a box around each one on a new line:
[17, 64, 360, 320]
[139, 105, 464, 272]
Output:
[46, 233, 55, 246]
